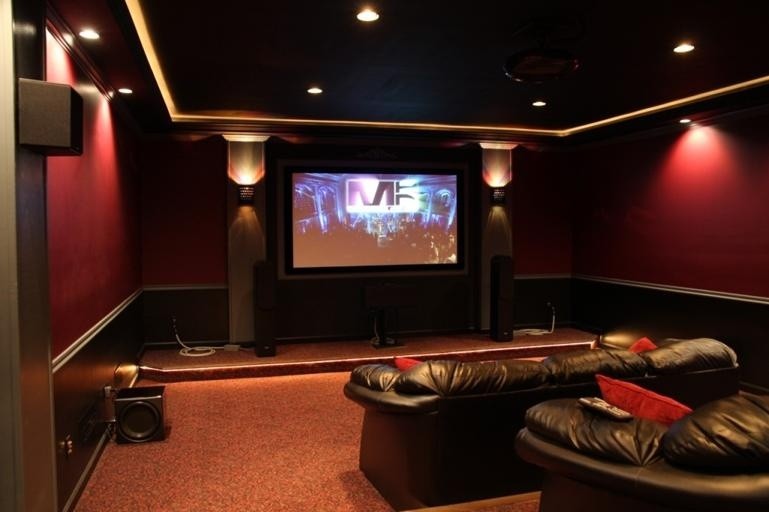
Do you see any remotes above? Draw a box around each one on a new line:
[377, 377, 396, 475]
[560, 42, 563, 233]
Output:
[578, 396, 634, 423]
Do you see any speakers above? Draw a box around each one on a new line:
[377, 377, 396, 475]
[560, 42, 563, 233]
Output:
[114, 385, 166, 444]
[255, 261, 276, 357]
[490, 255, 513, 341]
[18, 77, 83, 156]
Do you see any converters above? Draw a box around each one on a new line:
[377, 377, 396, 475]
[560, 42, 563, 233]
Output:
[224, 344, 241, 352]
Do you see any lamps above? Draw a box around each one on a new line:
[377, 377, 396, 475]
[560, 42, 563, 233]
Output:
[236, 184, 258, 205]
[489, 190, 506, 203]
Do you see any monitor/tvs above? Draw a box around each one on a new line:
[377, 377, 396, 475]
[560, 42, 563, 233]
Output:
[283, 166, 465, 276]
